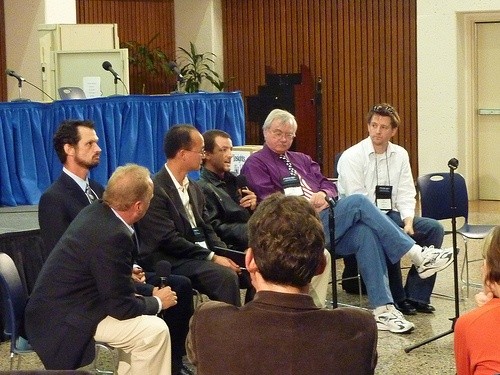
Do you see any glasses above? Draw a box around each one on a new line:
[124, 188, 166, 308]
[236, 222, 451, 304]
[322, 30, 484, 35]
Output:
[372, 105, 393, 114]
[186, 148, 207, 157]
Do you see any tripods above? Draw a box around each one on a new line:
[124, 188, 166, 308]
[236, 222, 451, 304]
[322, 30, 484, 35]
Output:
[404, 178, 460, 355]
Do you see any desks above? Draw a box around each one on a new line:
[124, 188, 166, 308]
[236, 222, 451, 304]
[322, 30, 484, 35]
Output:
[0, 90, 247, 208]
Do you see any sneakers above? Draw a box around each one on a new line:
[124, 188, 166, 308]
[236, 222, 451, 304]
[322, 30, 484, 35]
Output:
[413, 245, 460, 280]
[371, 305, 415, 333]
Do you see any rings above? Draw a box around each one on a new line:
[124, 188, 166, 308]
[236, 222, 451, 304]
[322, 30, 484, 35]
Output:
[249, 200, 251, 205]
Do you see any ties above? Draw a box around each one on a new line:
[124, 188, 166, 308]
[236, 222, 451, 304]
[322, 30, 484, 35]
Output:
[85, 183, 98, 204]
[280, 153, 298, 178]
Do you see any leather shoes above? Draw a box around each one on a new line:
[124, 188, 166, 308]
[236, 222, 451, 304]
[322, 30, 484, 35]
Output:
[393, 299, 435, 314]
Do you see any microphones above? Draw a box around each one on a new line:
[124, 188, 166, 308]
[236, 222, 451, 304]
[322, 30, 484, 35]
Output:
[448, 158, 458, 177]
[157, 260, 171, 318]
[102, 61, 121, 82]
[169, 61, 185, 82]
[325, 195, 336, 208]
[4, 68, 25, 81]
[236, 175, 250, 209]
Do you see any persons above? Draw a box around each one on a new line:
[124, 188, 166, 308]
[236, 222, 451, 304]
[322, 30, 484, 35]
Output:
[20, 163, 171, 375]
[452, 223, 500, 375]
[241, 108, 460, 334]
[196, 129, 259, 250]
[186, 189, 379, 375]
[135, 123, 259, 309]
[339, 102, 445, 314]
[38, 119, 195, 375]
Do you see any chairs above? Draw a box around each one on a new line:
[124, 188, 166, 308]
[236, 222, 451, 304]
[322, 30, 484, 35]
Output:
[0, 251, 117, 375]
[417, 172, 497, 301]
